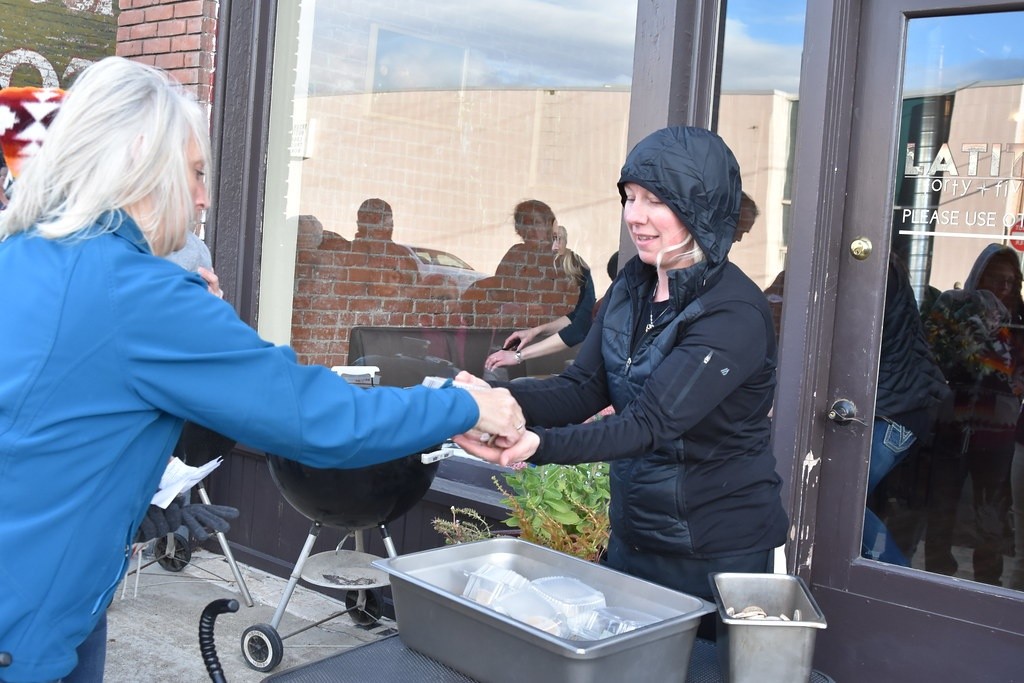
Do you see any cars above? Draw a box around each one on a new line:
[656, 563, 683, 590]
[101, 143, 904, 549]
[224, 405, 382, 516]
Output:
[409, 244, 484, 293]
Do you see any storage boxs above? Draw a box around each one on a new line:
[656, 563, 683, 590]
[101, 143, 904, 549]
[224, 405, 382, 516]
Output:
[371, 534, 828, 682]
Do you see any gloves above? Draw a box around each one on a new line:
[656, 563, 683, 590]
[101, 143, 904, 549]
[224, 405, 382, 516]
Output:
[134, 488, 184, 544]
[181, 503, 240, 539]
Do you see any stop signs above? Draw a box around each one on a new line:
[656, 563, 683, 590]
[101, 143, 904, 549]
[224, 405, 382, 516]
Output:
[1009, 219, 1024, 253]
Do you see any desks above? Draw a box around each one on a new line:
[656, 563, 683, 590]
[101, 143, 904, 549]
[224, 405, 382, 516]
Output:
[259, 629, 836, 682]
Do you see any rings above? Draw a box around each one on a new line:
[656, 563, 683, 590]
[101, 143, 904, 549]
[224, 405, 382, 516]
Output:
[516, 423, 524, 431]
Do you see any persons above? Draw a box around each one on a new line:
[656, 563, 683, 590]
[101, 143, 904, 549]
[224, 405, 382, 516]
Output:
[450, 125, 791, 646]
[484, 211, 597, 372]
[0, 56, 527, 680]
[161, 233, 225, 306]
[925, 242, 1024, 587]
[292, 198, 582, 332]
[764, 253, 952, 567]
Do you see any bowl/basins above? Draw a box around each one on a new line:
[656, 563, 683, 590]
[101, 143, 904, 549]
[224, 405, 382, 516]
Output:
[462, 561, 664, 643]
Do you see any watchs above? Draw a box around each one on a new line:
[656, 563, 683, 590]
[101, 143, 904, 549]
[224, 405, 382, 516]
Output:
[515, 350, 521, 365]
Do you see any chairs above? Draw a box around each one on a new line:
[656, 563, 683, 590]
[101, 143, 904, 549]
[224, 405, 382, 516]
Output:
[121, 444, 258, 607]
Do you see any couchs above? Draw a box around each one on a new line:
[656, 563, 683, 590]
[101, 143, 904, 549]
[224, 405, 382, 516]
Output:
[348, 325, 586, 391]
